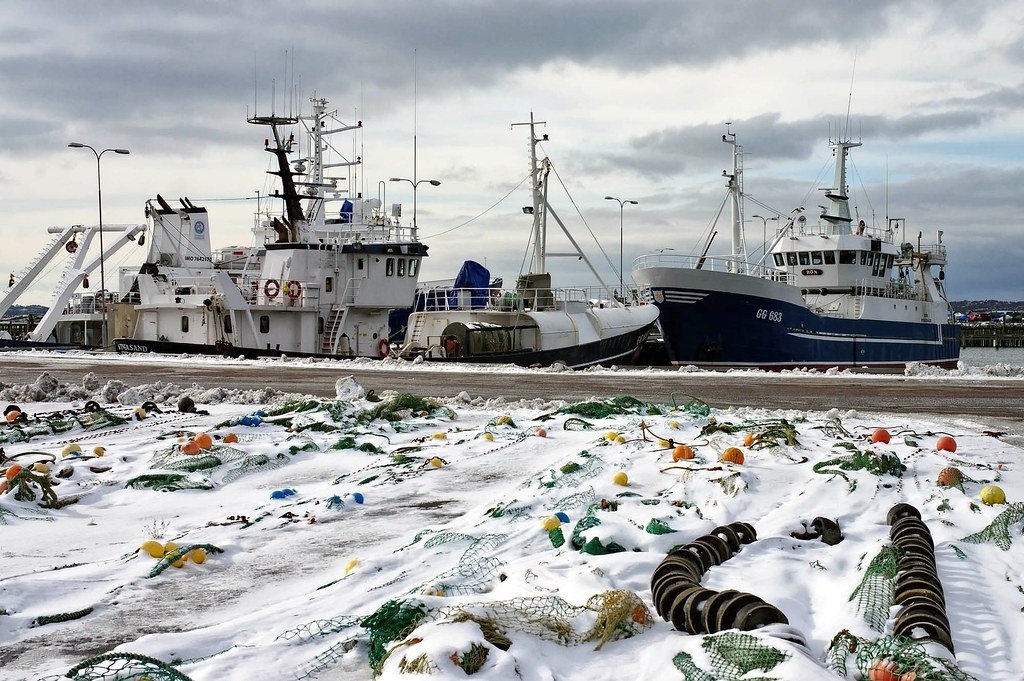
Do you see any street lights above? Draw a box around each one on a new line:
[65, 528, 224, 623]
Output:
[66, 142, 131, 351]
[604, 196, 638, 297]
[389, 178, 441, 241]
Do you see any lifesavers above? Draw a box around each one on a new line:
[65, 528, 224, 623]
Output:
[379, 339, 390, 356]
[287, 281, 302, 298]
[492, 290, 502, 297]
[264, 280, 280, 298]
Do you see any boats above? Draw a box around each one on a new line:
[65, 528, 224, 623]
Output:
[394, 109, 662, 369]
[627, 41, 966, 377]
[0, 49, 505, 363]
